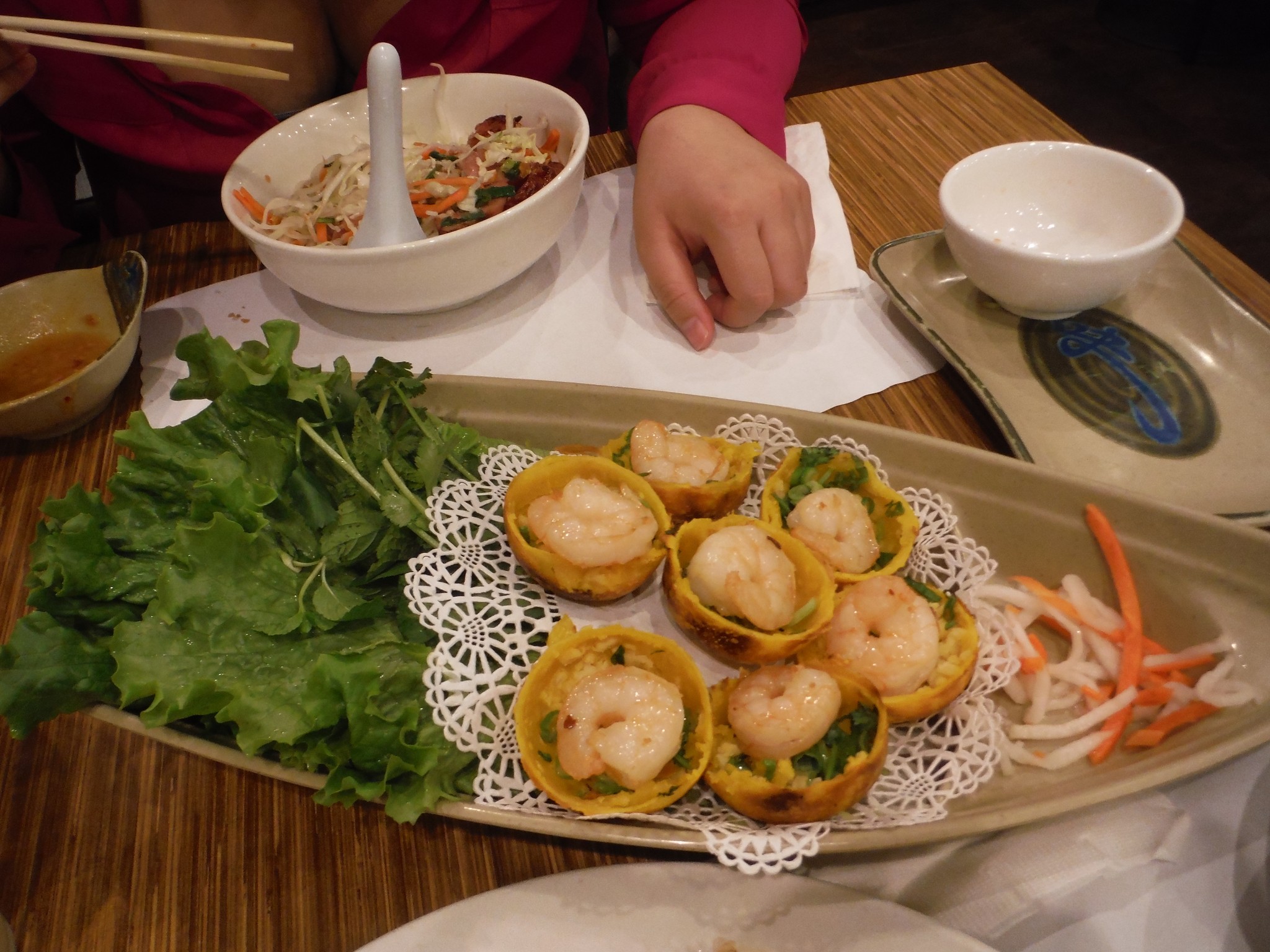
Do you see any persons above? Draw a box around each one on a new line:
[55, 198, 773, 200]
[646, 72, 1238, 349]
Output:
[0, 0, 816, 350]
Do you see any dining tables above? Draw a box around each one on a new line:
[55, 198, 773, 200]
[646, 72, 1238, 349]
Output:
[0, 60, 1269, 949]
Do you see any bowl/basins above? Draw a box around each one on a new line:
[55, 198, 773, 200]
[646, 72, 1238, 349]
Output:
[223, 74, 592, 315]
[940, 141, 1183, 319]
[1, 250, 149, 445]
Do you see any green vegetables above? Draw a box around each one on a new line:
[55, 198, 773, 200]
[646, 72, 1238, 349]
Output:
[0, 320, 594, 824]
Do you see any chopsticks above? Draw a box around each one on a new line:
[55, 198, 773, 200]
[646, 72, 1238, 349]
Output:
[0, 14, 294, 80]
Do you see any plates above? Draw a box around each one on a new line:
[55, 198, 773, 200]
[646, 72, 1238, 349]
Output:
[868, 226, 1269, 530]
[351, 861, 1001, 952]
[83, 374, 1270, 857]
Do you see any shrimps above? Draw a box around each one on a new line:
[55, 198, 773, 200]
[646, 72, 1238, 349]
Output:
[685, 524, 798, 631]
[554, 667, 686, 788]
[725, 664, 842, 760]
[786, 487, 880, 574]
[823, 576, 940, 696]
[630, 419, 729, 490]
[529, 474, 660, 571]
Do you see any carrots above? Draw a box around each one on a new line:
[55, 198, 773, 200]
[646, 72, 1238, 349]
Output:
[998, 501, 1235, 763]
[233, 124, 562, 247]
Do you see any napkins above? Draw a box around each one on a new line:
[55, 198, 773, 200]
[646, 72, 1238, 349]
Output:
[138, 120, 946, 430]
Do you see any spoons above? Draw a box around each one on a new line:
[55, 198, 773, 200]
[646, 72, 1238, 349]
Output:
[347, 41, 425, 249]
